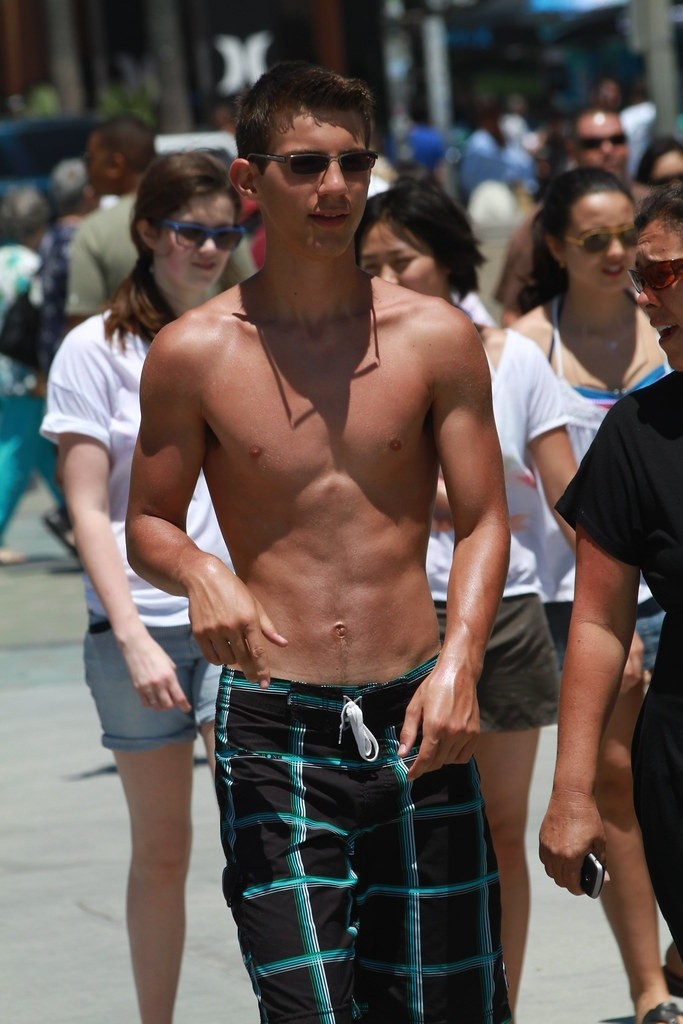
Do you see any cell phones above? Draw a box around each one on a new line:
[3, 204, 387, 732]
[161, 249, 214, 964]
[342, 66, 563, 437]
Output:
[581, 853, 605, 899]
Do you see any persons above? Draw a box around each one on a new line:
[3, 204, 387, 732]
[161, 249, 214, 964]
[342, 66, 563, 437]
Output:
[40, 136, 683, 1024]
[534, 178, 683, 965]
[126, 60, 512, 1024]
[0, 75, 683, 565]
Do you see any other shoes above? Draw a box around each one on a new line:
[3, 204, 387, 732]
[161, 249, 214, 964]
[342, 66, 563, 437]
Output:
[1, 542, 26, 567]
[42, 505, 84, 571]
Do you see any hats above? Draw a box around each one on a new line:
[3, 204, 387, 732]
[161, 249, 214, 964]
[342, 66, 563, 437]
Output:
[2, 184, 47, 235]
[48, 159, 87, 198]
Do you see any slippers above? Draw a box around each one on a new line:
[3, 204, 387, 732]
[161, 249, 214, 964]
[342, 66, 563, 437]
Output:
[661, 964, 683, 998]
[642, 1002, 683, 1024]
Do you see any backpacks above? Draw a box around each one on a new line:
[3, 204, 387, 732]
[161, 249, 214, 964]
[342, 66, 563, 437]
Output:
[35, 226, 72, 374]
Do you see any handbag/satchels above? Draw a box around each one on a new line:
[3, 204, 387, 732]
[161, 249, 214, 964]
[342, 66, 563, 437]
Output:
[0, 266, 43, 373]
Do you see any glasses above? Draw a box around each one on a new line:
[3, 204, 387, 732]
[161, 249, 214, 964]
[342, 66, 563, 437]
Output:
[627, 257, 683, 294]
[557, 228, 641, 254]
[579, 132, 628, 149]
[247, 150, 380, 175]
[163, 218, 245, 251]
